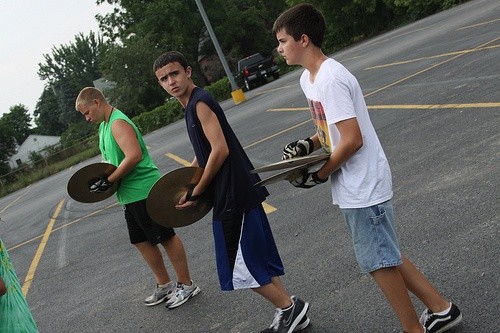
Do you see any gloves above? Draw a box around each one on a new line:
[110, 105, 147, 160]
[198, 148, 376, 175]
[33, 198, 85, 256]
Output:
[281, 138, 316, 160]
[89, 177, 113, 194]
[289, 172, 329, 189]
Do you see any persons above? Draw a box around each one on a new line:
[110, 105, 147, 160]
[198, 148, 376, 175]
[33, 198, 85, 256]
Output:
[272, 3, 463, 333]
[153, 51, 310, 333]
[75, 87, 200, 310]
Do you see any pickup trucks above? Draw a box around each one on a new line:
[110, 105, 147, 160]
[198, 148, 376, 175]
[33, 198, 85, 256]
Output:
[237, 53, 280, 90]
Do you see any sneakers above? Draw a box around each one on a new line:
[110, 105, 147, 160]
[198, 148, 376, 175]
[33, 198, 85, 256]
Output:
[418, 301, 463, 333]
[164, 279, 202, 308]
[261, 295, 313, 333]
[144, 281, 174, 306]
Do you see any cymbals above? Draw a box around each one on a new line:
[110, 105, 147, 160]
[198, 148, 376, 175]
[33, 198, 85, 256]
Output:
[249, 154, 331, 187]
[146, 166, 214, 227]
[68, 163, 120, 203]
